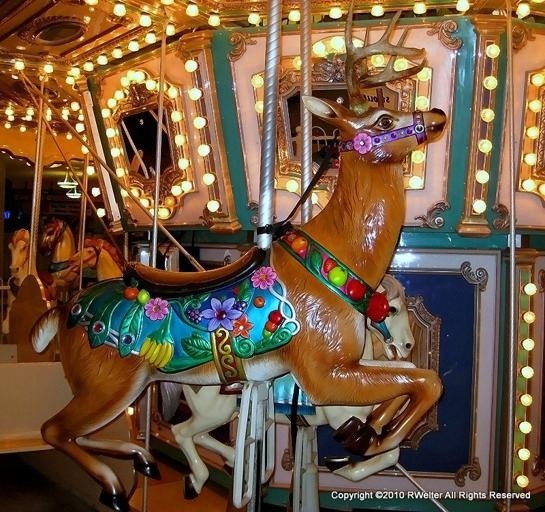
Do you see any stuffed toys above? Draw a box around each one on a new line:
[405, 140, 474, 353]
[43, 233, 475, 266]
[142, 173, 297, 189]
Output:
[0, 1, 449, 511]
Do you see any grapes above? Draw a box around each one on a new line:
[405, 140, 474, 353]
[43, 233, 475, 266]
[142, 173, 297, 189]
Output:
[233, 300, 247, 311]
[185, 308, 203, 324]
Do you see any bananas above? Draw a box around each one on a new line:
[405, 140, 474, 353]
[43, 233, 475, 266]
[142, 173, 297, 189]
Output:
[139, 306, 174, 368]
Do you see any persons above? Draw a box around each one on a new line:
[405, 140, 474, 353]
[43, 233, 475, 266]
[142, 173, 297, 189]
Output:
[0, 1, 449, 511]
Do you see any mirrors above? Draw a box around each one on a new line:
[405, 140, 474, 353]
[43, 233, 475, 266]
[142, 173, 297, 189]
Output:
[281, 83, 399, 177]
[119, 102, 175, 177]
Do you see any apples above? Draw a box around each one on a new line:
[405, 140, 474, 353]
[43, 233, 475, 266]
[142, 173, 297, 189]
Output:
[347, 279, 366, 301]
[329, 266, 348, 287]
[124, 285, 139, 299]
[137, 289, 151, 306]
[263, 310, 283, 339]
[324, 258, 338, 272]
[234, 287, 239, 294]
[254, 296, 264, 308]
